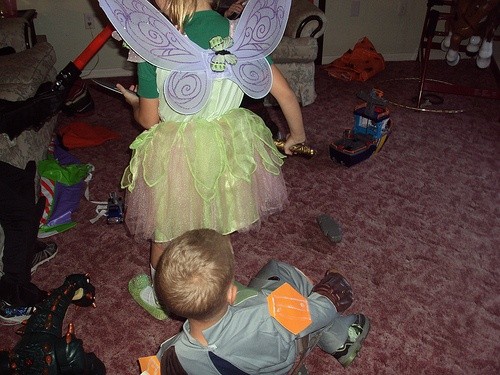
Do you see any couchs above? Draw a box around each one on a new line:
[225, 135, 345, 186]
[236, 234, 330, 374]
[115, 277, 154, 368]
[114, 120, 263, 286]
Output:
[263, 0, 328, 108]
[0, 18, 63, 277]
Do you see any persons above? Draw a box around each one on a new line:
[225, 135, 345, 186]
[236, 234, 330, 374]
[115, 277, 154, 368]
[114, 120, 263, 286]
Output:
[98, 0, 307, 320]
[0, 81, 59, 326]
[154, 228, 370, 375]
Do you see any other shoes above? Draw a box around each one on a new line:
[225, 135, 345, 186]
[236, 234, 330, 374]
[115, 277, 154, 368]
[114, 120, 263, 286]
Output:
[107, 192, 123, 223]
[129, 275, 167, 320]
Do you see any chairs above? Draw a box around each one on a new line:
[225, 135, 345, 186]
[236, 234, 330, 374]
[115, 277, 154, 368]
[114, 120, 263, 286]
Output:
[414, 0, 500, 113]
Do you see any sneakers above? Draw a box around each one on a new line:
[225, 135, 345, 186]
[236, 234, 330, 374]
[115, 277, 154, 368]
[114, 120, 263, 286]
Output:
[333, 313, 370, 367]
[0, 301, 38, 325]
[30, 242, 58, 274]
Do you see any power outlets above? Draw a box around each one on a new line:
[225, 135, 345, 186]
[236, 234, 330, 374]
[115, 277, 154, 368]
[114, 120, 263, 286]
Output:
[84, 14, 95, 29]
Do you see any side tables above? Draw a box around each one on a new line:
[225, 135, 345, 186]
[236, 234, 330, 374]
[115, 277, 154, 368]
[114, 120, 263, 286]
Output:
[18, 8, 39, 48]
